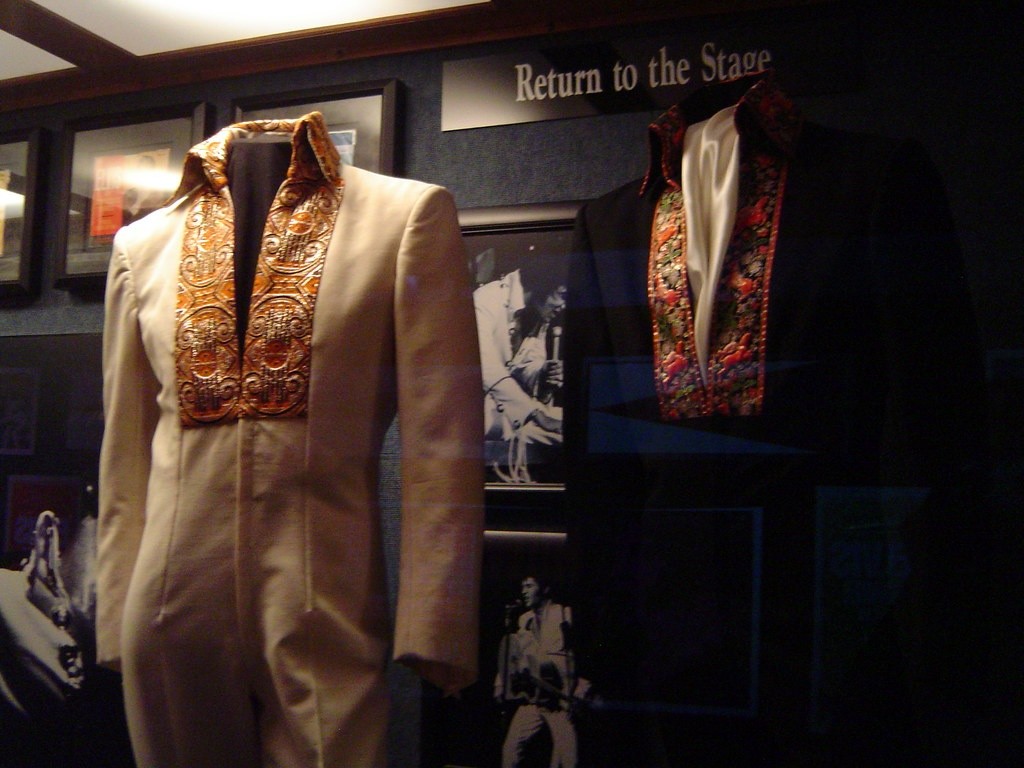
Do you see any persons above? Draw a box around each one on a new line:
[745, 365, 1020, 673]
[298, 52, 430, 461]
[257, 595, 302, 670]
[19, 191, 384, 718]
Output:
[472, 258, 563, 449]
[0, 478, 100, 768]
[498, 564, 591, 768]
[562, 66, 850, 768]
[122, 154, 168, 229]
[97, 109, 485, 768]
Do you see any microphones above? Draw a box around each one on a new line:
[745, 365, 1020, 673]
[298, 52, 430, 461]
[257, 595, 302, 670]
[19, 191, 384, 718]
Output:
[553, 326, 562, 361]
